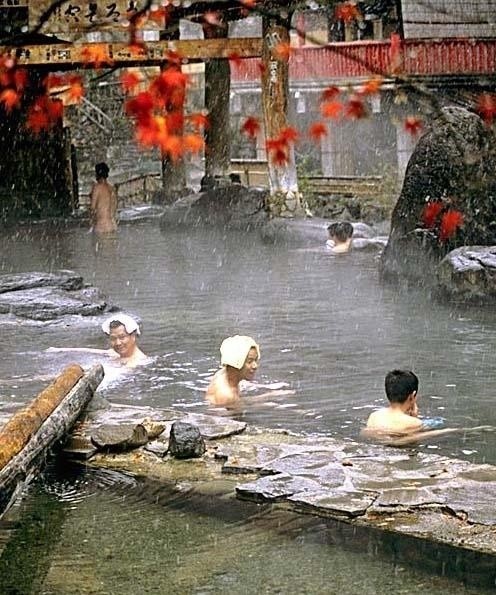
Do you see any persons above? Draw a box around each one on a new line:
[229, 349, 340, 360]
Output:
[90, 163, 118, 233]
[44, 314, 149, 367]
[326, 222, 354, 254]
[205, 335, 296, 413]
[359, 370, 496, 446]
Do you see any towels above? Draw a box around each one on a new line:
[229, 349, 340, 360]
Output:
[218, 334, 261, 370]
[103, 313, 141, 337]
